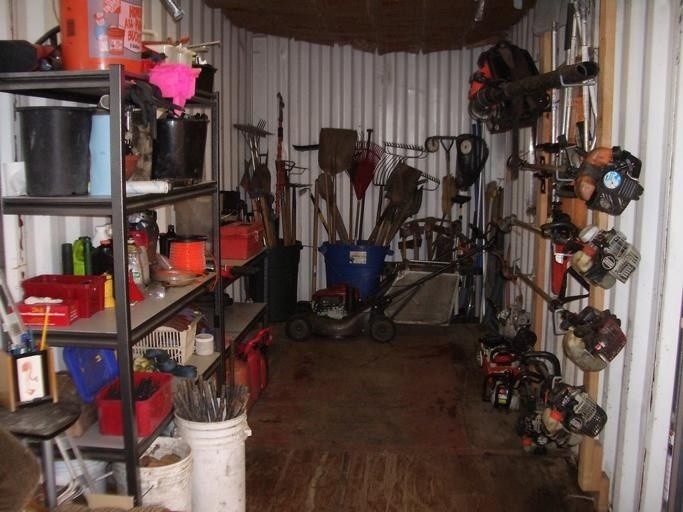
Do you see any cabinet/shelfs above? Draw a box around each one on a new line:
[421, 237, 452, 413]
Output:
[222, 246, 270, 398]
[0, 62, 224, 506]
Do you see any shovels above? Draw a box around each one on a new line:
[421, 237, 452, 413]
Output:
[249, 162, 278, 248]
[318, 127, 358, 244]
[366, 163, 423, 246]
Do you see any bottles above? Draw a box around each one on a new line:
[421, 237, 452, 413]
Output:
[61, 210, 178, 306]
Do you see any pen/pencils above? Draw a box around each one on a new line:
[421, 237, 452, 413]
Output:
[7, 324, 36, 358]
[39, 306, 50, 352]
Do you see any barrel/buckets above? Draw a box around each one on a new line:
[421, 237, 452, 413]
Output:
[153, 117, 210, 184]
[110, 437, 194, 512]
[61, 0, 144, 77]
[37, 458, 108, 493]
[121, 105, 156, 181]
[174, 398, 252, 512]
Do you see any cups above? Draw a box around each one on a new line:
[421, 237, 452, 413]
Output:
[196, 334, 214, 356]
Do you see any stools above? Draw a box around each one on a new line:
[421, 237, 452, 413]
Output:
[0, 402, 99, 506]
